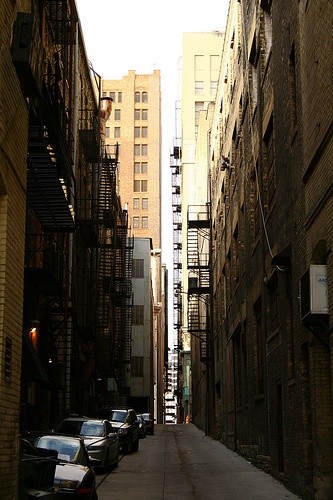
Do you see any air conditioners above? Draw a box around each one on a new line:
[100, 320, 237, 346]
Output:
[298, 264, 330, 321]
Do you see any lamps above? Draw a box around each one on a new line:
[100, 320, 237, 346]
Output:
[271, 254, 290, 271]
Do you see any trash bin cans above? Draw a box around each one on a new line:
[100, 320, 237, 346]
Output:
[18, 446, 58, 500]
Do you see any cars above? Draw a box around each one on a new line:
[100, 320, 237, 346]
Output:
[18, 433, 96, 500]
[45, 409, 156, 475]
